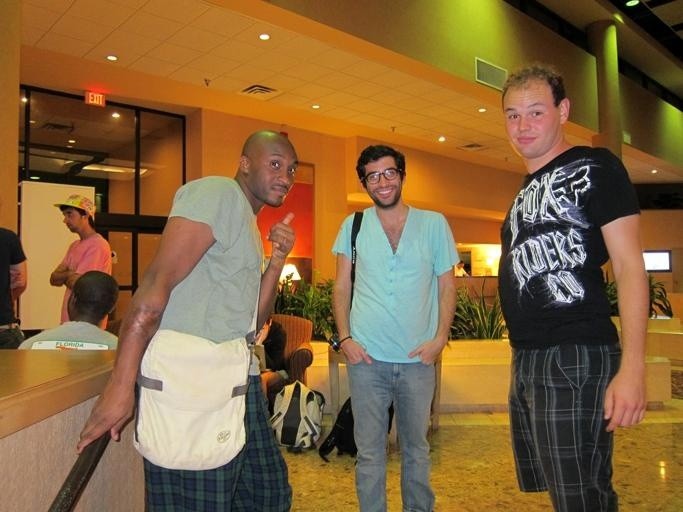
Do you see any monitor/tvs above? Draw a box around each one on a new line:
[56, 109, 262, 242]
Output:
[643, 250, 672, 273]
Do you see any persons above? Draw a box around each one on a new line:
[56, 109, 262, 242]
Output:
[454, 260, 470, 277]
[252, 317, 289, 400]
[49, 195, 113, 331]
[18, 270, 121, 350]
[498, 60, 651, 511]
[0, 226, 28, 349]
[75, 129, 293, 511]
[332, 144, 461, 512]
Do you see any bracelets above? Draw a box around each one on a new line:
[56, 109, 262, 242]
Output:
[337, 335, 352, 346]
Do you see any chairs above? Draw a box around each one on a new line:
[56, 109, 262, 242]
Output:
[262, 313, 314, 388]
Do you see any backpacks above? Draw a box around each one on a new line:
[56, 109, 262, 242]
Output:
[270, 380, 325, 452]
[320, 397, 394, 461]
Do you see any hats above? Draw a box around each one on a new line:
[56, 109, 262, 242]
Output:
[54, 194, 95, 217]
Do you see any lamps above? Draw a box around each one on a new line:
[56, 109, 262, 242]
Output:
[280, 263, 302, 291]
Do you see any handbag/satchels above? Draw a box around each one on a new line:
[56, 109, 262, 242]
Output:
[133, 330, 251, 471]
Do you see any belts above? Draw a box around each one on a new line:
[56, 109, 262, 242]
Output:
[0, 323, 20, 331]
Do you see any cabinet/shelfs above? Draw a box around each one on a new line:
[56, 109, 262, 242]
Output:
[1, 349, 145, 510]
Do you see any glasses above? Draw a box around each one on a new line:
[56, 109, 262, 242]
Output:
[365, 168, 401, 184]
